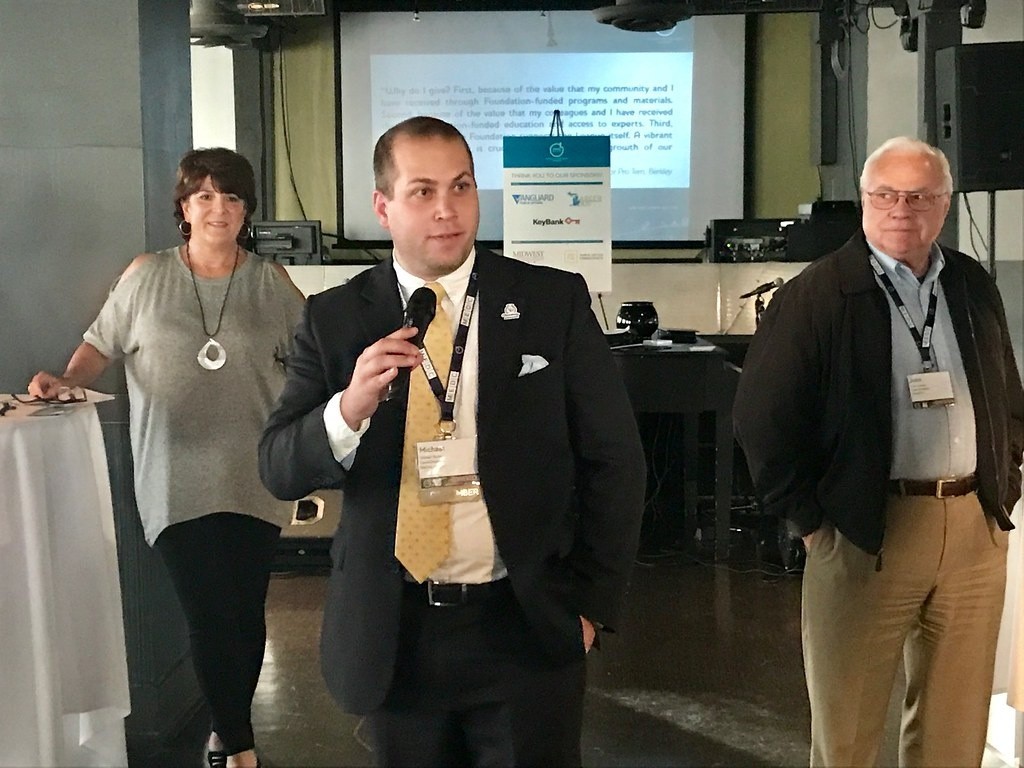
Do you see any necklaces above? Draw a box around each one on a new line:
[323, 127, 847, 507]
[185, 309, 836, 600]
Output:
[187, 240, 240, 371]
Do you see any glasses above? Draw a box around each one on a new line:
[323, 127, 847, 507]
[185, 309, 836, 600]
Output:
[865, 187, 946, 212]
[11, 386, 87, 403]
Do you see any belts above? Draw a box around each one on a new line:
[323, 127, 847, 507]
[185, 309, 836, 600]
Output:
[889, 475, 981, 498]
[401, 576, 510, 604]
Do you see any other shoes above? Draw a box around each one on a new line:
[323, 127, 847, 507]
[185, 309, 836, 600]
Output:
[208, 750, 223, 768]
[224, 756, 261, 768]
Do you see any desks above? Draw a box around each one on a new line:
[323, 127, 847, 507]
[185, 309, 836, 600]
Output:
[612, 337, 739, 561]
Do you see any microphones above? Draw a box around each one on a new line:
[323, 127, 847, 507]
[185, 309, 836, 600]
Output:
[386, 288, 437, 406]
[740, 277, 784, 299]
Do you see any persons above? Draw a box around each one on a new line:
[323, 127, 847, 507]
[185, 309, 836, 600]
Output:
[259, 117, 647, 768]
[27, 149, 306, 768]
[732, 136, 1024, 768]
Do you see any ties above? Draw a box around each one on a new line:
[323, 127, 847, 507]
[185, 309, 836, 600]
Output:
[394, 283, 455, 583]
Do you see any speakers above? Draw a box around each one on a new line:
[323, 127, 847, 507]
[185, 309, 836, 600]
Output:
[934, 41, 1024, 193]
